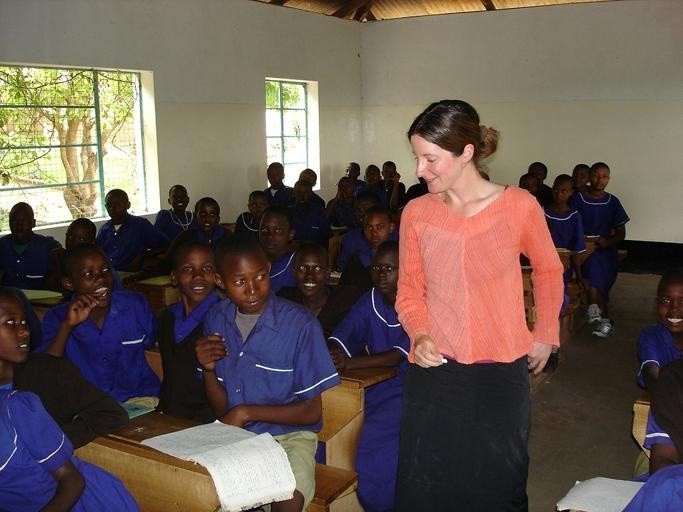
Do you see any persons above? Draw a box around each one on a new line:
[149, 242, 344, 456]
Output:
[394, 99, 566, 512]
[1, 160, 683, 511]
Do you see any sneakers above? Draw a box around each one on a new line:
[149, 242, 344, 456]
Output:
[587, 303, 601, 324]
[591, 318, 616, 339]
